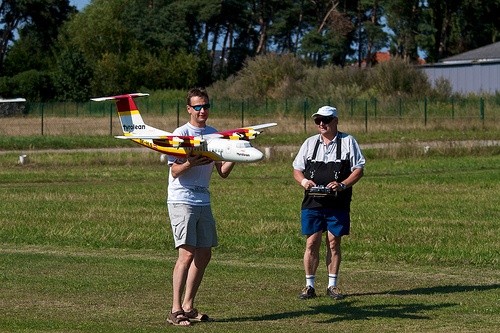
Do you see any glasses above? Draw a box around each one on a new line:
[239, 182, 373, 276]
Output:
[314, 116, 336, 125]
[191, 104, 210, 111]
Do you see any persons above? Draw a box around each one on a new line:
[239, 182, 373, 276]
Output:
[168, 88, 237, 326]
[291, 106, 366, 300]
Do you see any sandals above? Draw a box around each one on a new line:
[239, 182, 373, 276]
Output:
[166, 309, 192, 326]
[185, 308, 213, 322]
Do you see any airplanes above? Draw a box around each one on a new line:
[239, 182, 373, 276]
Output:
[90, 92, 278, 163]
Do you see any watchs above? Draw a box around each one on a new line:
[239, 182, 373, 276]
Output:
[339, 183, 345, 190]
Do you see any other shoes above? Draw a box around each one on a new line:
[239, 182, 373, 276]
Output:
[300, 286, 316, 298]
[327, 286, 343, 300]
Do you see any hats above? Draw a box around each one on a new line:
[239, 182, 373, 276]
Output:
[310, 106, 337, 118]
